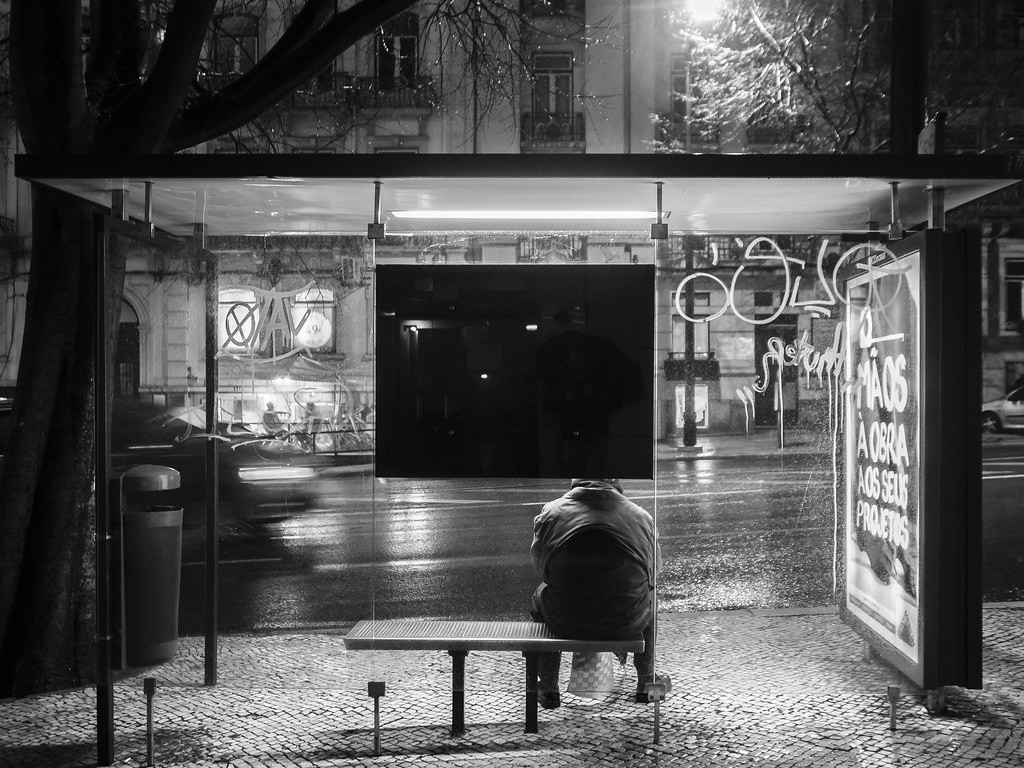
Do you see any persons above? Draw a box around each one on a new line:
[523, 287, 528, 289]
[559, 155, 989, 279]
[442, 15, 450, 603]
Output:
[295, 402, 314, 450]
[529, 479, 670, 709]
[263, 402, 280, 428]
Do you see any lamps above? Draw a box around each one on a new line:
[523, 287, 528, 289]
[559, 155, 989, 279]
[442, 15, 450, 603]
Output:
[888, 182, 905, 242]
[140, 181, 155, 239]
[367, 181, 386, 239]
[650, 182, 669, 239]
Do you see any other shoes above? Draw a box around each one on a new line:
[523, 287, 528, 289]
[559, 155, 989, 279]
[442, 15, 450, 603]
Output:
[537, 680, 561, 709]
[635, 673, 672, 703]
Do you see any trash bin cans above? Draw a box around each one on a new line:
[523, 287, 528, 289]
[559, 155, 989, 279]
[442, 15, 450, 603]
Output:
[107, 464, 184, 667]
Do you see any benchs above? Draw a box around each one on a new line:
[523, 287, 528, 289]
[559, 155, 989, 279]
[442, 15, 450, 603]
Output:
[343, 620, 646, 734]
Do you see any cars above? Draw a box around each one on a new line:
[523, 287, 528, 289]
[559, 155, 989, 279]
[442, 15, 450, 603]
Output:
[111, 399, 321, 530]
[982, 383, 1024, 433]
